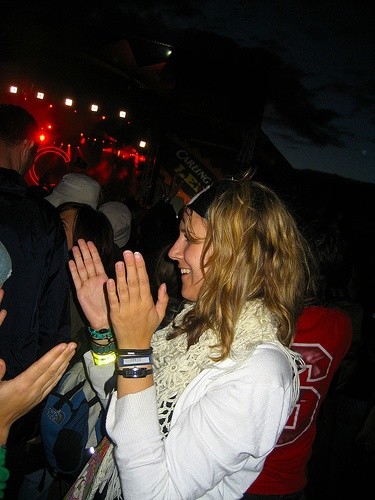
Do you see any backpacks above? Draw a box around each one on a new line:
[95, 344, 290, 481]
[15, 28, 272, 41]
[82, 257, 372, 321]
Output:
[40, 378, 103, 477]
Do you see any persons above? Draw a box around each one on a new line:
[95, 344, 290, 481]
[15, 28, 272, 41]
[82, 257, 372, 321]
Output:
[0, 98, 375, 500]
[62, 174, 309, 499]
[0, 288, 78, 500]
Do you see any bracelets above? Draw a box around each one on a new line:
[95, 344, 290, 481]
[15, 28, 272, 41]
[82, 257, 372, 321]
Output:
[116, 367, 154, 378]
[116, 355, 154, 366]
[90, 341, 118, 354]
[88, 327, 116, 340]
[116, 347, 152, 354]
[92, 354, 116, 366]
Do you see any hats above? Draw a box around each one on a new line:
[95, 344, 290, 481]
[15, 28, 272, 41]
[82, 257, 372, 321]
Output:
[44, 173, 100, 210]
[98, 202, 131, 246]
